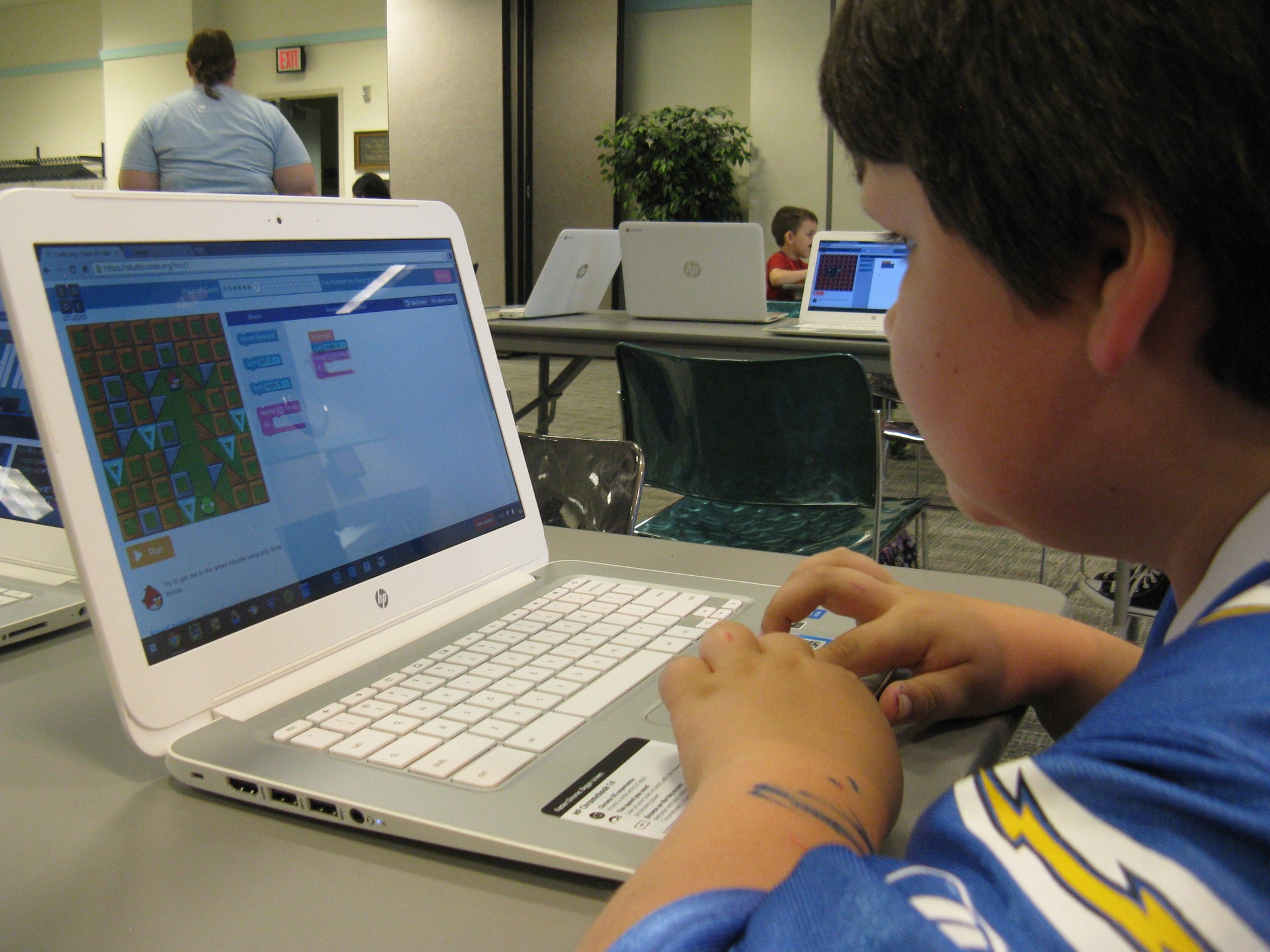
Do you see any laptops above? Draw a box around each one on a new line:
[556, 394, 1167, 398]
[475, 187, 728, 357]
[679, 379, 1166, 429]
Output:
[0, 185, 913, 888]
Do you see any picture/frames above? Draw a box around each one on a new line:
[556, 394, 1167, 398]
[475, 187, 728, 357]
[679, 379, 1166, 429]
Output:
[353, 129, 390, 171]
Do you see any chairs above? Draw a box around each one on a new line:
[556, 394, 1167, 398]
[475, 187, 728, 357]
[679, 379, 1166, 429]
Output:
[615, 337, 935, 568]
[515, 432, 646, 539]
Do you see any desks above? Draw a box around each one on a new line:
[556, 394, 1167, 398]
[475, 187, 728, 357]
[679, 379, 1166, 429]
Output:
[0, 525, 1069, 952]
[483, 304, 894, 507]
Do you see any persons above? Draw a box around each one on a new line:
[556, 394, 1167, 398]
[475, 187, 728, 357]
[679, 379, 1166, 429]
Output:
[766, 206, 819, 301]
[581, 0, 1270, 952]
[352, 172, 391, 198]
[118, 28, 318, 196]
